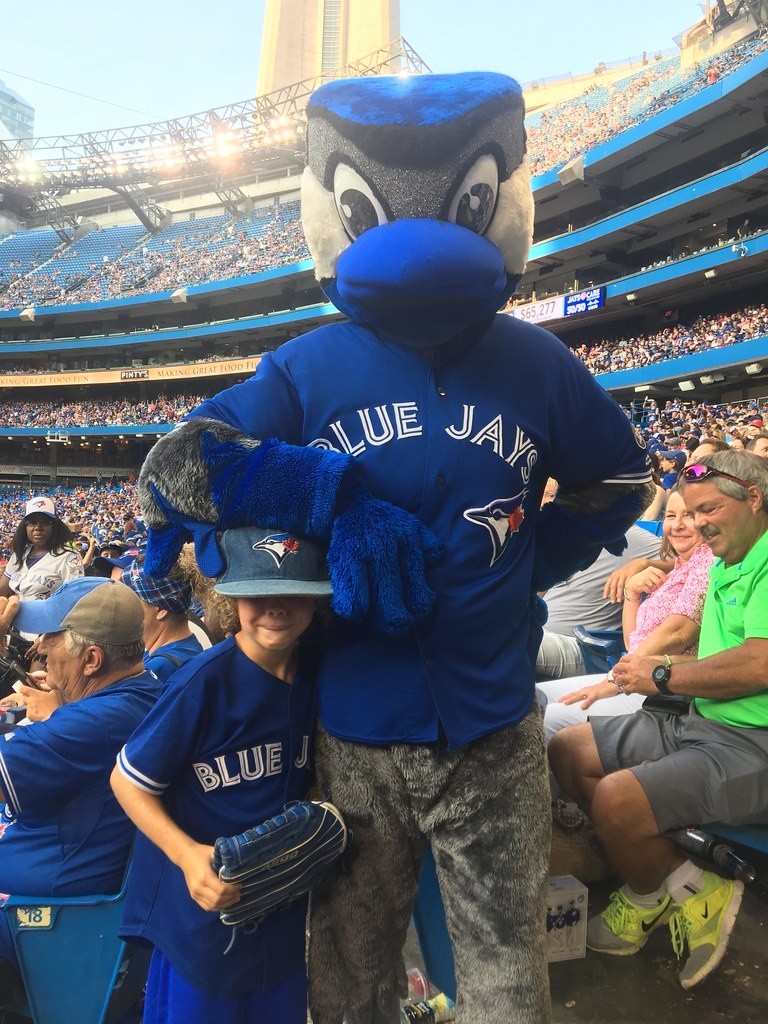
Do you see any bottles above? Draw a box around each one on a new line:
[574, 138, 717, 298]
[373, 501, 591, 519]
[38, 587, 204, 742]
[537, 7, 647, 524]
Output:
[400, 992, 457, 1024]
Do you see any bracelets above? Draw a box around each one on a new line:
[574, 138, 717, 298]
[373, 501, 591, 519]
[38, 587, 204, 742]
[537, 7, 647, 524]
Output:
[623, 587, 643, 602]
[665, 653, 672, 666]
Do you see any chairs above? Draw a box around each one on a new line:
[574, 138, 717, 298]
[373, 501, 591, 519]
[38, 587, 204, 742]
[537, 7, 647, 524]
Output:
[635, 519, 768, 856]
[1, 814, 457, 1024]
[0, 482, 137, 505]
[523, 38, 768, 178]
[0, 199, 310, 310]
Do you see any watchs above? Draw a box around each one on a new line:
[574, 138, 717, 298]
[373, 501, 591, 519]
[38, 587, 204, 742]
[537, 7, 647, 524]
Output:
[608, 670, 626, 692]
[652, 661, 673, 694]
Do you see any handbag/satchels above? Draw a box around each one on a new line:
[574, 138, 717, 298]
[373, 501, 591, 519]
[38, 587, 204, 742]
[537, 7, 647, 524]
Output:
[549, 797, 600, 880]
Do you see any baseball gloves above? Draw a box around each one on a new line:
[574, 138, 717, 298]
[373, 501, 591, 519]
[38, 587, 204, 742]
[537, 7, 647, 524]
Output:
[211, 800, 354, 927]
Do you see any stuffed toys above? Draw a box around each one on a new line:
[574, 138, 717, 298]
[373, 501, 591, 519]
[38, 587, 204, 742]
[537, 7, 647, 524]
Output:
[142, 71, 660, 1024]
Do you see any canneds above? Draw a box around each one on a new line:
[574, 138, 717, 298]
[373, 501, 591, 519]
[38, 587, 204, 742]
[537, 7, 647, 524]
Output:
[0, 701, 17, 717]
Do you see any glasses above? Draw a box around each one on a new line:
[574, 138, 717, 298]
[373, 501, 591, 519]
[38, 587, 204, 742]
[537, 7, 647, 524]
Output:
[23, 518, 54, 528]
[676, 463, 752, 490]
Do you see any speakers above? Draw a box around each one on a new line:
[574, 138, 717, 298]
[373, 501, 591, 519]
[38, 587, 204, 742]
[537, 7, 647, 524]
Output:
[704, 270, 717, 279]
[19, 309, 34, 322]
[679, 380, 695, 391]
[626, 293, 637, 301]
[170, 288, 187, 304]
[745, 363, 763, 375]
[558, 156, 585, 186]
[700, 375, 715, 385]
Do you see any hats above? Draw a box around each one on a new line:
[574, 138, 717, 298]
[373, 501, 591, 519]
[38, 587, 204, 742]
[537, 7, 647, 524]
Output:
[11, 576, 143, 646]
[642, 397, 760, 467]
[213, 526, 334, 598]
[94, 547, 147, 574]
[110, 526, 148, 546]
[24, 496, 57, 520]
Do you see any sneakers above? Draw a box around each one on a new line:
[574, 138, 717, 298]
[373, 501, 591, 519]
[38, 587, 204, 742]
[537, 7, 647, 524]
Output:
[667, 871, 746, 990]
[586, 883, 675, 954]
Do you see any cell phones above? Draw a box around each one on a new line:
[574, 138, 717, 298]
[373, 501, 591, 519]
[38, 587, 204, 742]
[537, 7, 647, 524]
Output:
[10, 662, 40, 692]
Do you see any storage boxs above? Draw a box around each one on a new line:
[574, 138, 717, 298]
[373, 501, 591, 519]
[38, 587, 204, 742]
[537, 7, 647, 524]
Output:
[545, 875, 588, 963]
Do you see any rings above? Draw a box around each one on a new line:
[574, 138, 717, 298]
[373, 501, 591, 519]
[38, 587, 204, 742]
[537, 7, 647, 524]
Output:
[648, 581, 653, 588]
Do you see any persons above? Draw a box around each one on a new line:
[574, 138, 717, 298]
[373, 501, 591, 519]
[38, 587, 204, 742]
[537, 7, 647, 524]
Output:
[1, 473, 144, 597]
[0, 358, 61, 375]
[0, 201, 310, 311]
[549, 453, 768, 992]
[535, 490, 721, 751]
[610, 389, 768, 490]
[193, 352, 237, 361]
[122, 572, 202, 684]
[0, 576, 165, 1024]
[110, 527, 346, 1024]
[7, 395, 221, 426]
[639, 219, 764, 272]
[561, 303, 767, 376]
[525, 0, 768, 179]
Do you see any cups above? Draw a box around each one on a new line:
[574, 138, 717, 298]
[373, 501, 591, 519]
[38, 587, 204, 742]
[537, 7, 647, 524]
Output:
[399, 966, 430, 1006]
